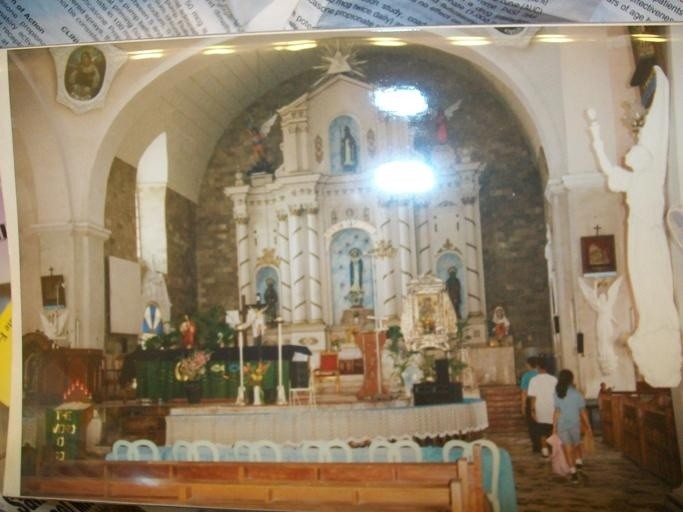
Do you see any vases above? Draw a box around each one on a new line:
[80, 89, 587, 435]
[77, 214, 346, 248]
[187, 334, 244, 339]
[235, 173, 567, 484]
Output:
[252, 385, 263, 405]
[185, 378, 203, 403]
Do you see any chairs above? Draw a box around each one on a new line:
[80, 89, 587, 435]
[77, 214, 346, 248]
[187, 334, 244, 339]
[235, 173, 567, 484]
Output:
[109, 431, 499, 510]
[288, 350, 341, 407]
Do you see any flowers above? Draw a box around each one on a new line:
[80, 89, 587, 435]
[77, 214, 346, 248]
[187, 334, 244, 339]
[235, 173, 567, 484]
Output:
[178, 351, 211, 380]
[243, 359, 270, 384]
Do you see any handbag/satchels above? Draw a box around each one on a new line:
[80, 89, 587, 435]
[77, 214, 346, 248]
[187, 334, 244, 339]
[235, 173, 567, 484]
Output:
[544, 433, 570, 478]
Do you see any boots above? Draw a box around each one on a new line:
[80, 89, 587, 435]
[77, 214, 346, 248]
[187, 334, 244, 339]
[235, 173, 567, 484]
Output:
[570, 462, 584, 484]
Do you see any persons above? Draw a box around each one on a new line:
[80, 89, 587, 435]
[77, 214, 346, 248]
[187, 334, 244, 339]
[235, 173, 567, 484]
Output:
[179, 314, 196, 349]
[69, 51, 100, 101]
[588, 122, 681, 388]
[244, 127, 271, 165]
[264, 278, 277, 324]
[593, 280, 619, 373]
[348, 248, 363, 292]
[520, 357, 593, 484]
[489, 306, 514, 347]
[341, 126, 356, 172]
[435, 108, 448, 143]
[445, 267, 462, 320]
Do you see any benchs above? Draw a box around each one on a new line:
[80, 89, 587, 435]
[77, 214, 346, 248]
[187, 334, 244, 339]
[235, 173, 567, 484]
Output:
[20, 443, 486, 511]
